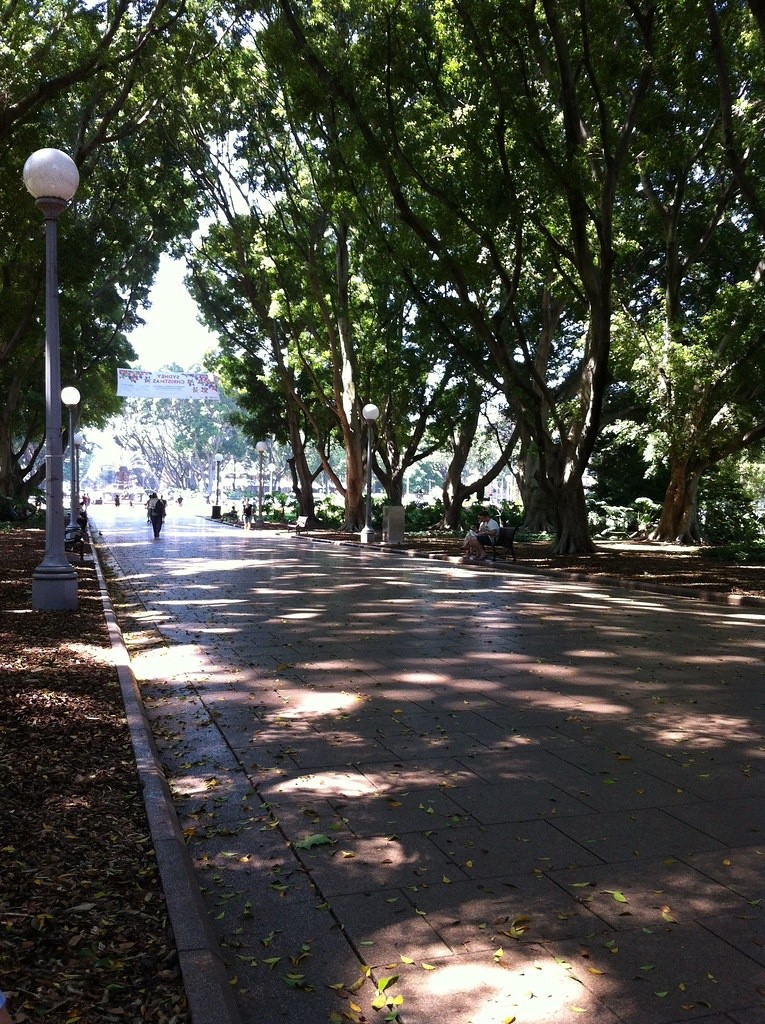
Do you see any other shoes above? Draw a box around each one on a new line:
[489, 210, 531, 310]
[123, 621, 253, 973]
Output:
[459, 545, 471, 550]
[477, 553, 488, 561]
[154, 532, 159, 538]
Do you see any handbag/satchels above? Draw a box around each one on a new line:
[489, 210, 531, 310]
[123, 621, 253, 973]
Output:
[153, 500, 164, 516]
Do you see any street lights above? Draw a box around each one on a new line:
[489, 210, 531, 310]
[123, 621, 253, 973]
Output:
[361, 403, 379, 543]
[256, 441, 267, 527]
[62, 387, 82, 541]
[25, 148, 79, 610]
[212, 453, 224, 518]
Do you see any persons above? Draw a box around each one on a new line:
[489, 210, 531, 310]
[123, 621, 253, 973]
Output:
[115, 495, 120, 506]
[460, 511, 499, 560]
[241, 497, 253, 529]
[144, 493, 166, 537]
[82, 493, 90, 510]
[222, 506, 236, 519]
[178, 495, 183, 503]
